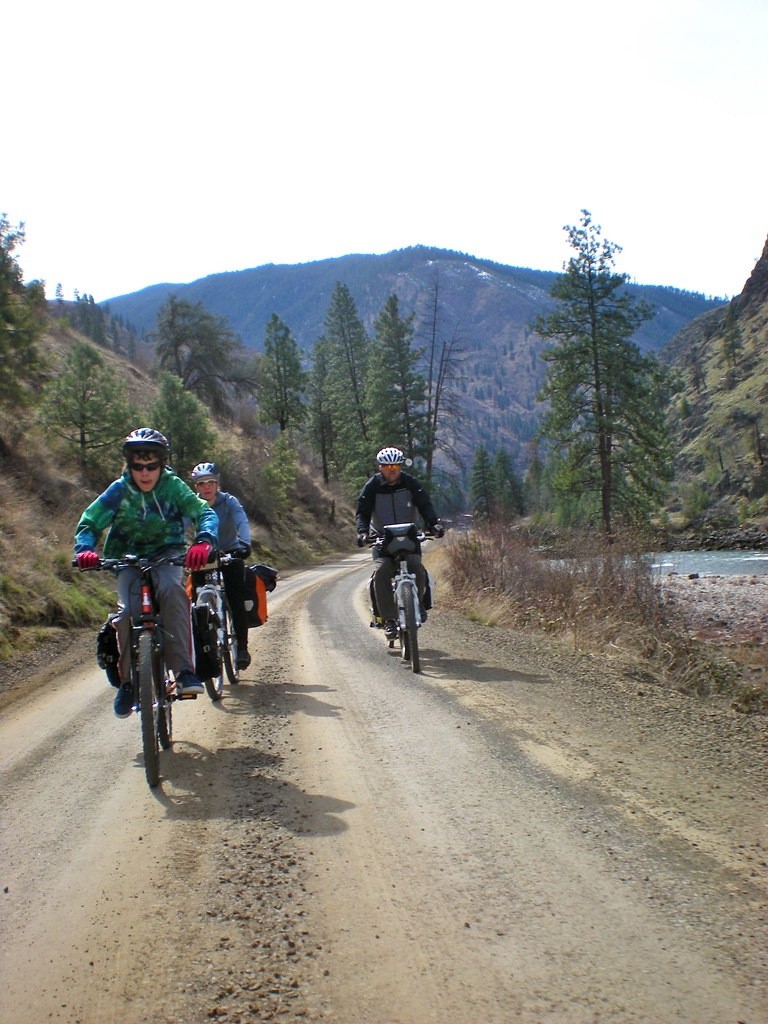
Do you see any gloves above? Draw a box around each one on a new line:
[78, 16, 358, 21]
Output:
[185, 540, 211, 571]
[356, 532, 368, 548]
[230, 548, 250, 559]
[76, 549, 97, 572]
[429, 523, 444, 539]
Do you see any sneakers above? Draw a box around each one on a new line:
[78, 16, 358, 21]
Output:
[176, 670, 204, 694]
[113, 682, 134, 719]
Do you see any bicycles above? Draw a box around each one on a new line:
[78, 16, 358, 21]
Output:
[358, 530, 445, 673]
[175, 541, 241, 701]
[70, 554, 213, 788]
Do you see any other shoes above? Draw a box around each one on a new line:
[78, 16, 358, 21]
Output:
[236, 650, 250, 669]
[420, 605, 426, 622]
[383, 620, 398, 639]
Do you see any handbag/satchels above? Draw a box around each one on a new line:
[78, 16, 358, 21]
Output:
[192, 603, 223, 680]
[96, 612, 122, 688]
[248, 564, 278, 629]
[424, 568, 434, 611]
[366, 575, 380, 617]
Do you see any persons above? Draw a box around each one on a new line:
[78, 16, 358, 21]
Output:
[72, 428, 204, 719]
[177, 462, 251, 669]
[357, 446, 444, 638]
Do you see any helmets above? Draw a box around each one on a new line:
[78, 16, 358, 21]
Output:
[377, 447, 405, 464]
[191, 461, 219, 485]
[121, 428, 170, 467]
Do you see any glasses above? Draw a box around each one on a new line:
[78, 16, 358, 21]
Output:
[380, 465, 400, 470]
[129, 461, 162, 471]
[197, 481, 216, 486]
